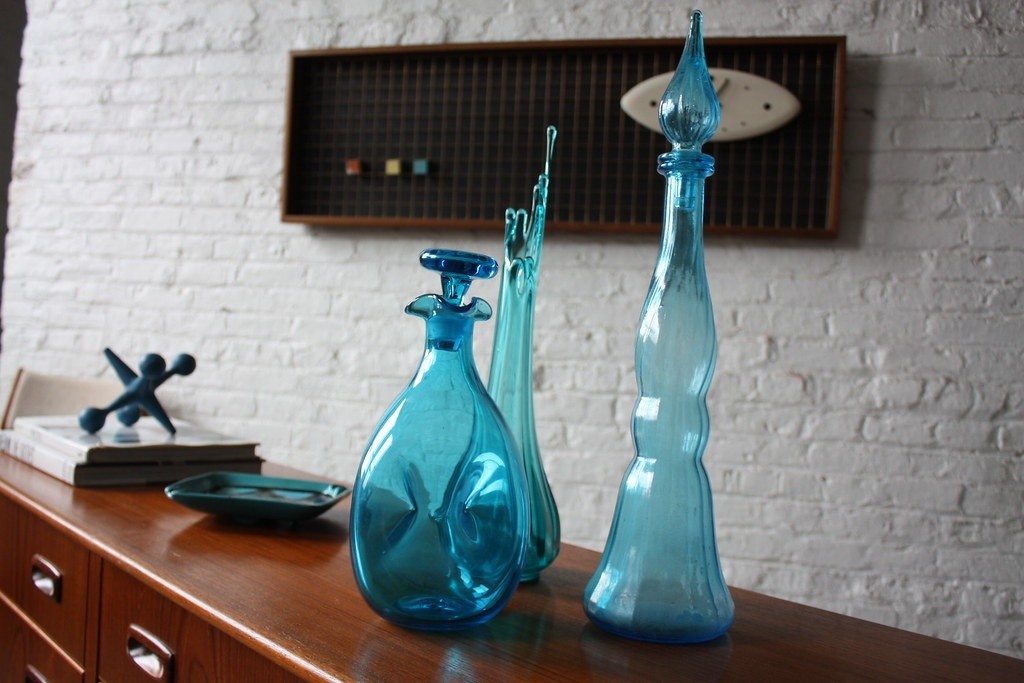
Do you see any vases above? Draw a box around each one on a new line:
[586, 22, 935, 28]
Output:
[350, 126, 561, 632]
[580, 11, 735, 645]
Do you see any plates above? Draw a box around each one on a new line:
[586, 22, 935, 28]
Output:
[165, 471, 351, 523]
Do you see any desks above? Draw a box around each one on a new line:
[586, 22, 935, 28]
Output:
[0, 448, 1024, 683]
